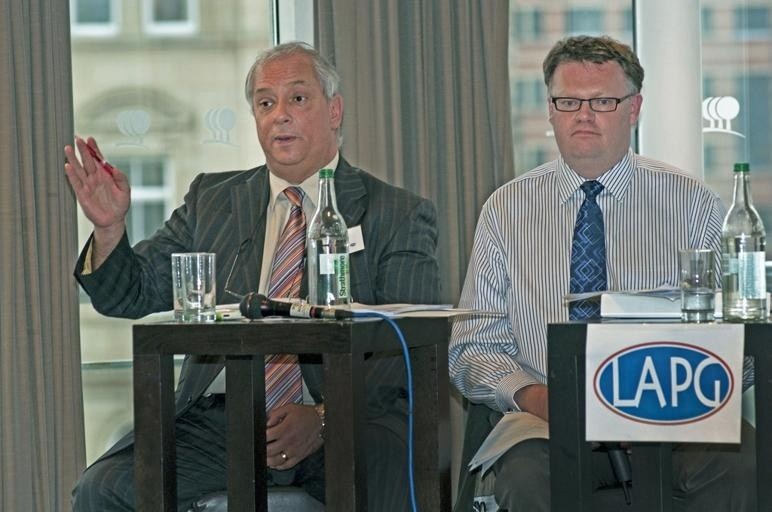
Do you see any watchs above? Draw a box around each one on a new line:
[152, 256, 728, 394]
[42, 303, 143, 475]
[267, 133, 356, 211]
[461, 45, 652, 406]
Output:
[315, 403, 329, 440]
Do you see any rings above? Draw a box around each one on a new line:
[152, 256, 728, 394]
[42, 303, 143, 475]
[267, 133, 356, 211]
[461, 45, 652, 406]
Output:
[280, 452, 288, 461]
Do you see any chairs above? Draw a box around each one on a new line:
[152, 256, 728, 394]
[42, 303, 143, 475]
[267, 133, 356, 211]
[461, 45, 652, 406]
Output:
[186, 483, 327, 512]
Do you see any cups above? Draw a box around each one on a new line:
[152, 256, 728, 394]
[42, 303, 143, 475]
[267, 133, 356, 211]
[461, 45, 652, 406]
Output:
[170, 251, 217, 323]
[677, 248, 717, 323]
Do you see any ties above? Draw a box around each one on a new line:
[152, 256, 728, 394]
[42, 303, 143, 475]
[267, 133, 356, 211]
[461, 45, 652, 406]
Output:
[568, 181, 608, 325]
[263, 187, 307, 419]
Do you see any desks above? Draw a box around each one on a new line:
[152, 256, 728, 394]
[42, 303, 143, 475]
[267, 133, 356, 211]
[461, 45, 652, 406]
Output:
[545, 317, 772, 512]
[130, 307, 451, 512]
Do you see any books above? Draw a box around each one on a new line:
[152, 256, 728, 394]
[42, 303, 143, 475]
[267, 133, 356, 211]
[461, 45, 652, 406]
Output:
[467, 411, 549, 478]
[335, 302, 453, 316]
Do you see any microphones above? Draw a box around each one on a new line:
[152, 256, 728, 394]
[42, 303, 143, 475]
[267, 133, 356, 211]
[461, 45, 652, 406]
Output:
[239, 292, 354, 320]
[600, 442, 633, 507]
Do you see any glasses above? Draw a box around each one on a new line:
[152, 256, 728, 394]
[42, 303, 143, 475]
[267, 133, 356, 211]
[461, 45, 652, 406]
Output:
[549, 91, 637, 113]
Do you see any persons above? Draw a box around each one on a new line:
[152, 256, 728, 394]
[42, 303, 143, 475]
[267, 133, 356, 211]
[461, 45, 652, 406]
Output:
[447, 35, 756, 512]
[63, 40, 441, 512]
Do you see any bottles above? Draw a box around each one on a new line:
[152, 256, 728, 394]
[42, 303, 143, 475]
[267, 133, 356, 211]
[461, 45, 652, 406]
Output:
[305, 168, 352, 307]
[720, 161, 767, 323]
